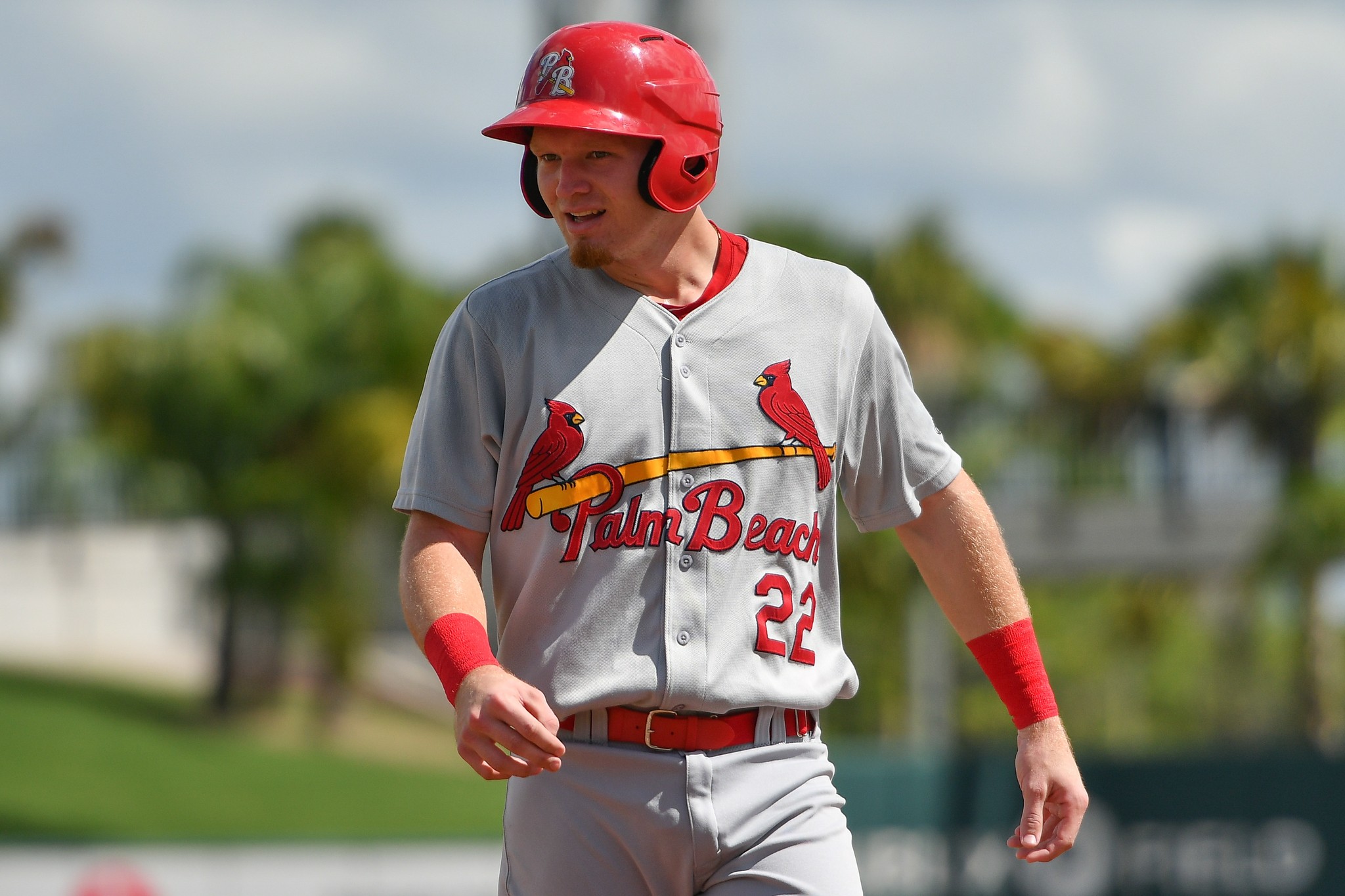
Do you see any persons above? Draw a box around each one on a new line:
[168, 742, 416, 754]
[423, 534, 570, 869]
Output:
[397, 22, 1091, 896]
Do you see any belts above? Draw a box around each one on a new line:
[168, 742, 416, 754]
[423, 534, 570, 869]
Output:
[559, 706, 814, 750]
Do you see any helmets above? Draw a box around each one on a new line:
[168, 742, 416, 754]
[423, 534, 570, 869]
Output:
[481, 21, 723, 219]
